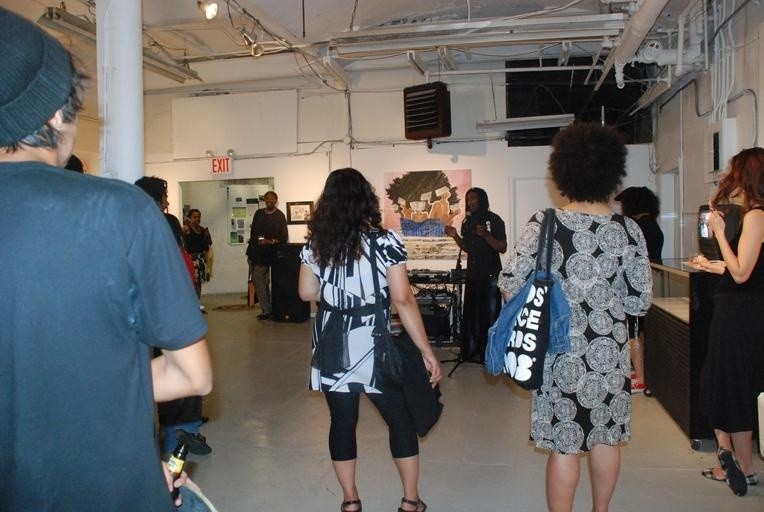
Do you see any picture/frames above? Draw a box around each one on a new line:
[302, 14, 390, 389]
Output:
[286, 201, 314, 224]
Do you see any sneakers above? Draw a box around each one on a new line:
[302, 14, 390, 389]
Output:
[174, 430, 212, 455]
[703, 446, 758, 497]
[631, 371, 645, 394]
[256, 314, 272, 321]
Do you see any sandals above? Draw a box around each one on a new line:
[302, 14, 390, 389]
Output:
[340, 499, 362, 512]
[398, 497, 427, 512]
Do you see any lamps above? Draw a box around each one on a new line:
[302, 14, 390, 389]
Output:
[475, 74, 576, 134]
[37, 0, 203, 84]
[198, 0, 218, 20]
[237, 28, 264, 56]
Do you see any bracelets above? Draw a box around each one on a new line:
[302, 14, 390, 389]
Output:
[271, 238, 276, 245]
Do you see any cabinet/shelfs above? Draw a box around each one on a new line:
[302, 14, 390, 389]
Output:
[644, 257, 758, 450]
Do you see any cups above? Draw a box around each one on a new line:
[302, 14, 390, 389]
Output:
[258, 238, 265, 247]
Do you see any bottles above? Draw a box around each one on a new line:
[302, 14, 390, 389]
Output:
[165, 442, 191, 501]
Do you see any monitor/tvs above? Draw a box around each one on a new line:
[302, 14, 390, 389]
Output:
[697, 204, 742, 260]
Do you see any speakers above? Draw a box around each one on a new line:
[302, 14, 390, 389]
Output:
[271, 243, 311, 323]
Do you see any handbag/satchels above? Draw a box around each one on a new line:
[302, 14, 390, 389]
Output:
[501, 278, 556, 390]
[373, 326, 403, 389]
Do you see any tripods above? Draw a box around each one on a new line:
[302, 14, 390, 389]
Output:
[438, 273, 486, 377]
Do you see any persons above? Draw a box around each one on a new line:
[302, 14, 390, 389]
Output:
[247, 191, 288, 321]
[2, 7, 212, 511]
[445, 188, 507, 363]
[615, 187, 665, 393]
[691, 147, 764, 496]
[497, 122, 654, 510]
[298, 168, 443, 512]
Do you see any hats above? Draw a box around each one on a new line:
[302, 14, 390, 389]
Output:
[0, 6, 75, 147]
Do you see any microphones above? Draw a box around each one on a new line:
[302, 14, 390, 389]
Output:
[461, 212, 472, 236]
